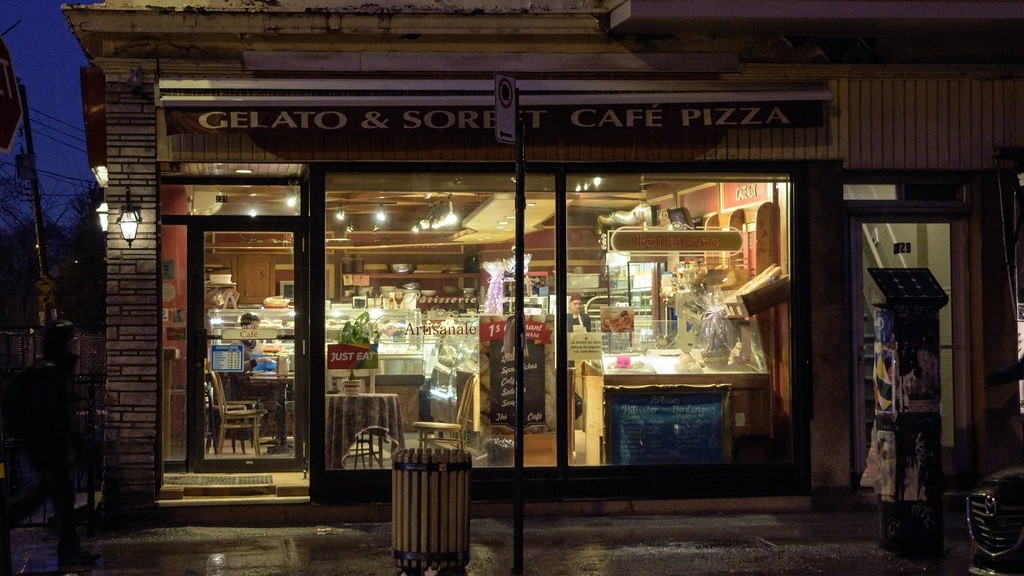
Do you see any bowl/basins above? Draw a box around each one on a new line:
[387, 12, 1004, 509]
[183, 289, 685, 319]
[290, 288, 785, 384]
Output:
[421, 289, 437, 296]
[392, 264, 414, 274]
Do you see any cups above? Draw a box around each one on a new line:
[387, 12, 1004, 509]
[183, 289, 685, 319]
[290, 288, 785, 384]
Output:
[379, 286, 397, 292]
[463, 288, 475, 298]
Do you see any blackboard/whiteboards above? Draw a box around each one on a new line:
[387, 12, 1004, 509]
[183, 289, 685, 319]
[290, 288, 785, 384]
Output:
[601, 383, 733, 466]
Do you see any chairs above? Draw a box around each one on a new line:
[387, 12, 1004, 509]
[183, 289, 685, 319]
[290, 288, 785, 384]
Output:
[410, 374, 475, 451]
[206, 358, 267, 456]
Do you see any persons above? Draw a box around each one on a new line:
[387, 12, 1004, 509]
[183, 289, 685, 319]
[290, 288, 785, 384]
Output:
[0, 319, 108, 566]
[566, 293, 591, 332]
[227, 313, 289, 444]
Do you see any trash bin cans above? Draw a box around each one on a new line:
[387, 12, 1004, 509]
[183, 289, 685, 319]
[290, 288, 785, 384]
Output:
[390, 448, 473, 576]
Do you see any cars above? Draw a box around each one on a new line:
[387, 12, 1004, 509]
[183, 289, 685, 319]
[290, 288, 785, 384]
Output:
[965, 468, 1024, 576]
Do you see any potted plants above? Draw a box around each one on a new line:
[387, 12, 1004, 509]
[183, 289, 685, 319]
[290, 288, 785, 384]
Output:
[337, 312, 381, 395]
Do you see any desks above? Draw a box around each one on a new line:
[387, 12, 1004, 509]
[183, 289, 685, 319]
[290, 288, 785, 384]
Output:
[324, 394, 406, 469]
[249, 372, 294, 452]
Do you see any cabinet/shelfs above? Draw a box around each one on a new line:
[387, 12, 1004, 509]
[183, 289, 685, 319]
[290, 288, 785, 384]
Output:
[342, 273, 480, 325]
[579, 353, 775, 467]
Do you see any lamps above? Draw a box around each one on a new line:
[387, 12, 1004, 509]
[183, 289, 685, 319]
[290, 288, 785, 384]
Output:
[417, 194, 460, 229]
[114, 187, 142, 249]
